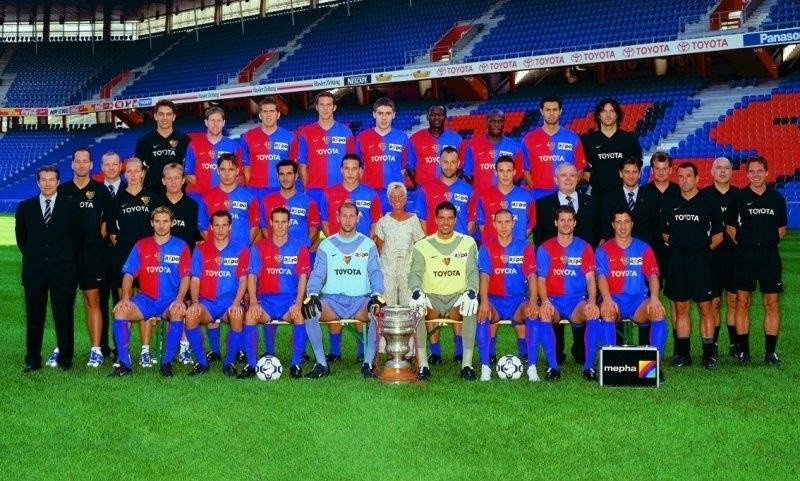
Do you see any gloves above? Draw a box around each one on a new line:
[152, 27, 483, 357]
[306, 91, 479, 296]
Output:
[367, 295, 386, 316]
[409, 290, 433, 316]
[453, 290, 479, 317]
[301, 295, 322, 320]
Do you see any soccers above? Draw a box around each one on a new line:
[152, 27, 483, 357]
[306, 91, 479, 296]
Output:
[255, 354, 282, 380]
[496, 354, 524, 380]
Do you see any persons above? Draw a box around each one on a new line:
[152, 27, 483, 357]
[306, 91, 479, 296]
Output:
[44, 147, 113, 370]
[699, 157, 746, 363]
[107, 88, 387, 380]
[355, 95, 686, 381]
[98, 151, 129, 366]
[14, 164, 80, 375]
[660, 162, 725, 373]
[724, 156, 789, 367]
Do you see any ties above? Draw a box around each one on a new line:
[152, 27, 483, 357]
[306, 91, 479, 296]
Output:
[108, 185, 115, 198]
[628, 192, 635, 213]
[43, 199, 52, 226]
[565, 196, 577, 215]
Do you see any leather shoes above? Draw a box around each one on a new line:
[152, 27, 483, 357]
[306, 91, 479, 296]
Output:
[25, 349, 70, 371]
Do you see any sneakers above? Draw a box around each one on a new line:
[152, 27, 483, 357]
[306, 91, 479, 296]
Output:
[356, 353, 377, 379]
[764, 353, 781, 365]
[88, 347, 275, 380]
[544, 368, 560, 382]
[480, 364, 492, 381]
[453, 353, 462, 364]
[583, 368, 598, 383]
[527, 364, 541, 382]
[702, 342, 718, 369]
[428, 354, 442, 365]
[489, 354, 497, 365]
[289, 351, 341, 378]
[417, 366, 430, 380]
[460, 366, 477, 381]
[518, 354, 528, 367]
[670, 353, 693, 367]
[729, 344, 751, 365]
